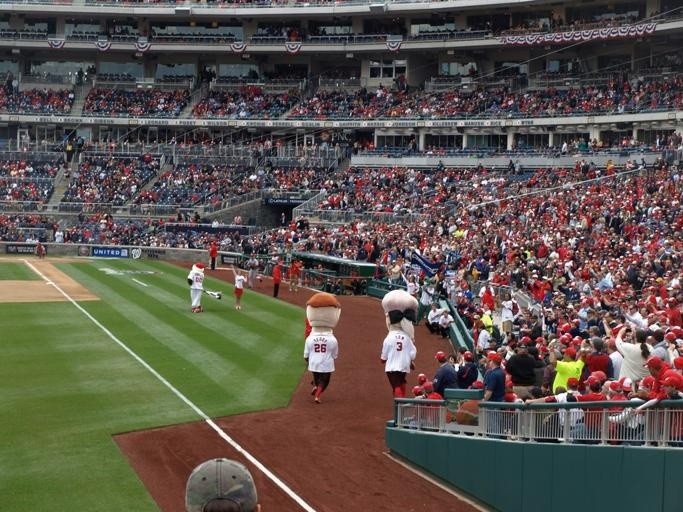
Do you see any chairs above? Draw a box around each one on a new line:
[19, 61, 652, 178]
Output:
[0, 1, 683, 444]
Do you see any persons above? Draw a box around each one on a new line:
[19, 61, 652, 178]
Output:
[34, 241, 46, 259]
[1, 65, 191, 118]
[120, 25, 157, 43]
[186, 0, 683, 449]
[1, 132, 186, 249]
[36, 28, 48, 40]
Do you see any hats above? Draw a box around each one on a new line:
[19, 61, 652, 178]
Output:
[410, 271, 683, 404]
